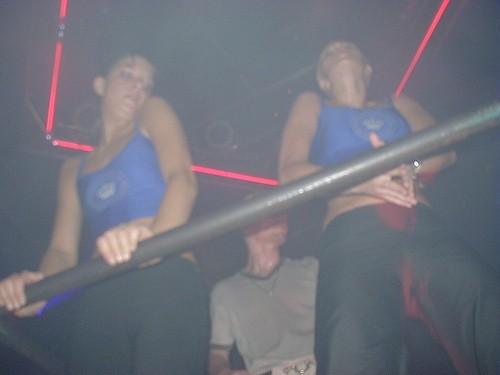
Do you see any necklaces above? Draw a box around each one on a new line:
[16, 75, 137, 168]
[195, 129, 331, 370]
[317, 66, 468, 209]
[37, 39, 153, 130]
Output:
[249, 277, 277, 296]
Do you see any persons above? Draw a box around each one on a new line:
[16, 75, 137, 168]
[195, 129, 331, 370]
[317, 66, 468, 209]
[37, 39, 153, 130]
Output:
[204, 190, 319, 375]
[277, 37, 500, 375]
[0, 43, 211, 375]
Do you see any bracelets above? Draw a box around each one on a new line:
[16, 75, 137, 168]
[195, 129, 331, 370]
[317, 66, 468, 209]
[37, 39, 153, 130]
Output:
[413, 160, 422, 173]
